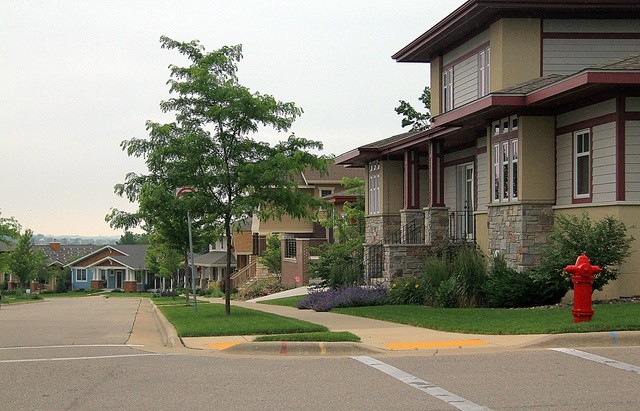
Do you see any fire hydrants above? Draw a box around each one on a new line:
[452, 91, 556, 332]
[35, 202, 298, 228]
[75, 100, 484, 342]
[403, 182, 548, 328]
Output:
[563, 250, 603, 322]
